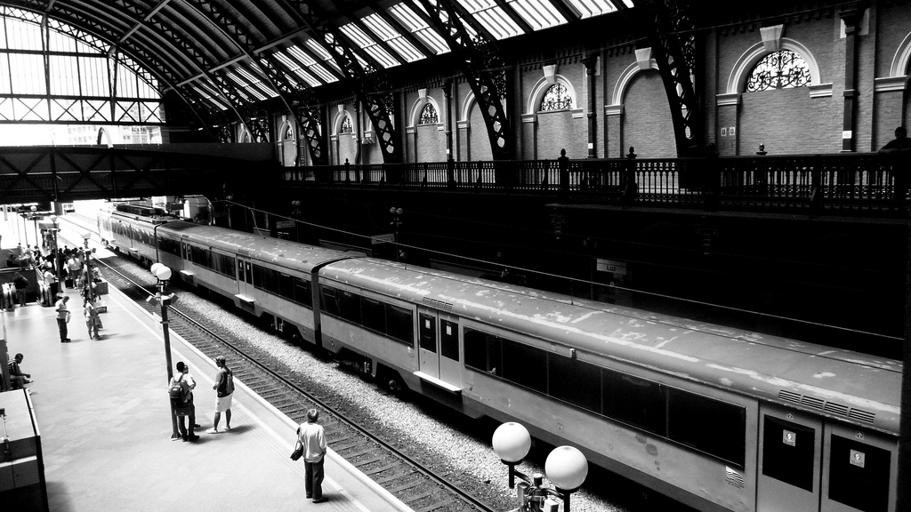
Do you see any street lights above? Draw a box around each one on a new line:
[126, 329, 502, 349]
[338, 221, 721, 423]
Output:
[490, 419, 590, 510]
[30, 205, 42, 249]
[79, 231, 98, 306]
[48, 214, 62, 292]
[145, 262, 182, 439]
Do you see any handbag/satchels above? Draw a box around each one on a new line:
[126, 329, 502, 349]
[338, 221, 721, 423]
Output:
[291, 441, 304, 460]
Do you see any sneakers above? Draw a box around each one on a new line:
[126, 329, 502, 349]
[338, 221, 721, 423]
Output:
[312, 497, 330, 503]
[181, 423, 230, 443]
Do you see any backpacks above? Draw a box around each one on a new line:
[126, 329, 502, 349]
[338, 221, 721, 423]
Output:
[223, 372, 235, 394]
[170, 374, 189, 410]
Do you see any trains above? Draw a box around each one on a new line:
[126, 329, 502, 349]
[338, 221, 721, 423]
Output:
[73, 193, 905, 511]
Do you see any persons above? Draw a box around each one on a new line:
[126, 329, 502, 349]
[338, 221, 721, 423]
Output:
[8, 353, 33, 384]
[55, 296, 72, 342]
[83, 295, 103, 340]
[182, 365, 201, 428]
[299, 409, 327, 503]
[168, 361, 201, 442]
[7, 243, 101, 306]
[208, 355, 235, 433]
